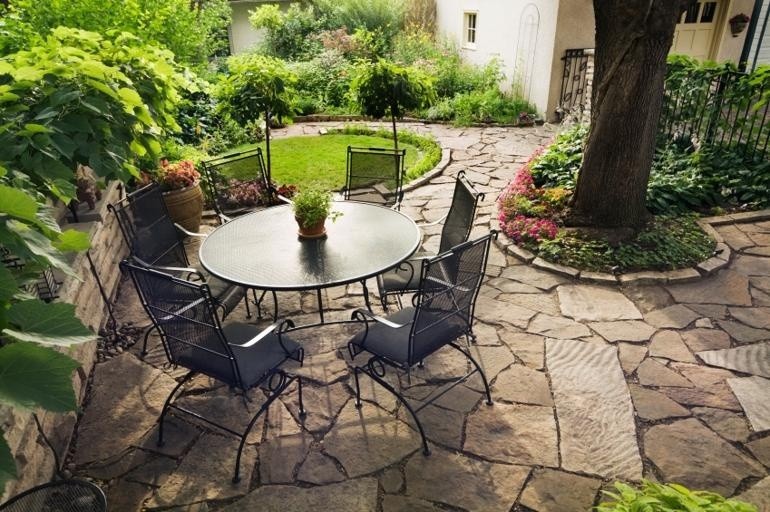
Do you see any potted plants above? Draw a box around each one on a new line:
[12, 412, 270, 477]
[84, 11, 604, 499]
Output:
[291, 178, 345, 239]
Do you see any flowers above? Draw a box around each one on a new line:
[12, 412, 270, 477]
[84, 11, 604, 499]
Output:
[135, 157, 201, 192]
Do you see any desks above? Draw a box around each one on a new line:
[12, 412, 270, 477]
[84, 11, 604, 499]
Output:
[199, 200, 422, 336]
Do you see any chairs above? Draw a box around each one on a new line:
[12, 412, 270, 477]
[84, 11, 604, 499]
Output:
[106, 179, 252, 320]
[376, 169, 485, 343]
[345, 227, 498, 458]
[121, 261, 306, 484]
[202, 146, 293, 224]
[333, 145, 405, 211]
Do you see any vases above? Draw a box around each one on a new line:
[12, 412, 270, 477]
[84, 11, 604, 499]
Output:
[162, 179, 204, 236]
